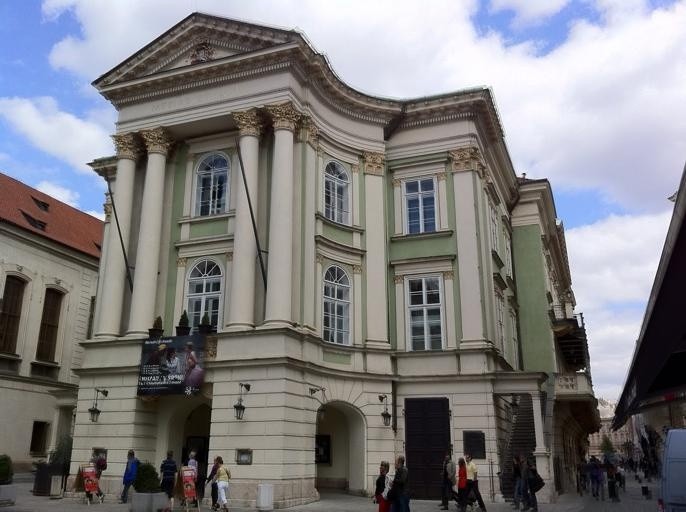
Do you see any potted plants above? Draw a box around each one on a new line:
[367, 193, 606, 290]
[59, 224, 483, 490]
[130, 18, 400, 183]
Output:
[197, 311, 213, 336]
[147, 314, 166, 337]
[0, 452, 18, 506]
[127, 458, 167, 511]
[174, 309, 192, 337]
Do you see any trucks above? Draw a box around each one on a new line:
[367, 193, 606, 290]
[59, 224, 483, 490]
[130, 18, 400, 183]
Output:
[658, 428, 686, 511]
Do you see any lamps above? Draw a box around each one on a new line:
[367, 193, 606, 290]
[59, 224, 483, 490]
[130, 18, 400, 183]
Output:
[233, 382, 251, 421]
[308, 386, 329, 421]
[378, 393, 391, 426]
[87, 386, 108, 424]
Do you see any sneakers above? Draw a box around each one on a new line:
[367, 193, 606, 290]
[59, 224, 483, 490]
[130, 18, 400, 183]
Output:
[439, 500, 539, 512]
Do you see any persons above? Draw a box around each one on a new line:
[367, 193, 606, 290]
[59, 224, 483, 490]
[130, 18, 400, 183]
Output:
[183, 341, 204, 388]
[210, 460, 231, 512]
[206, 456, 222, 510]
[373, 461, 394, 512]
[518, 452, 538, 512]
[180, 451, 198, 507]
[387, 455, 412, 512]
[118, 450, 140, 504]
[159, 450, 179, 512]
[575, 454, 626, 502]
[512, 454, 528, 509]
[165, 346, 180, 375]
[85, 452, 105, 503]
[440, 453, 486, 512]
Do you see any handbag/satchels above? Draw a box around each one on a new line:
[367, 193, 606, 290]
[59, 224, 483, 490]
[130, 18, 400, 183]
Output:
[527, 469, 546, 494]
